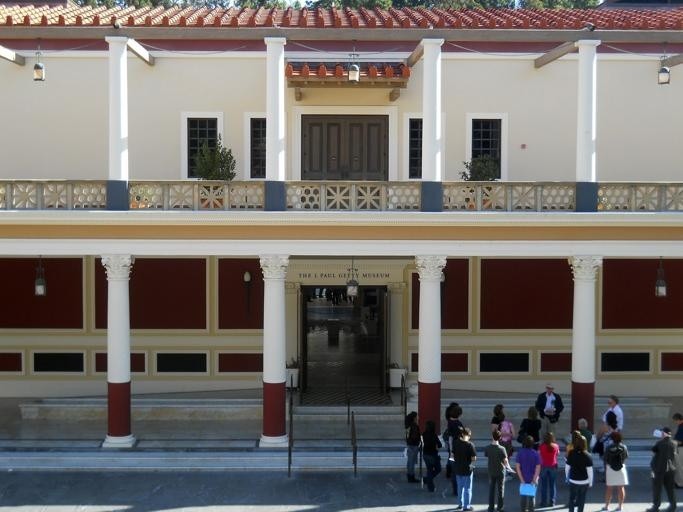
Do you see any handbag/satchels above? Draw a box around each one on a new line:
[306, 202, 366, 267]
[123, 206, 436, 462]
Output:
[609, 447, 624, 471]
[594, 442, 604, 456]
[518, 483, 538, 498]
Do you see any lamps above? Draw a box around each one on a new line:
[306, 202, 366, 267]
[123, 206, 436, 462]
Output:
[34, 254, 48, 295]
[653, 40, 671, 84]
[30, 42, 46, 81]
[344, 256, 361, 305]
[647, 255, 669, 298]
[239, 270, 254, 316]
[348, 39, 361, 85]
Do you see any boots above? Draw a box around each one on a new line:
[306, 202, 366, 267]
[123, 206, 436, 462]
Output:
[407, 474, 420, 483]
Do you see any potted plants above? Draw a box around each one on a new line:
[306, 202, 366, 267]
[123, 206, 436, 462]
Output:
[460, 151, 493, 211]
[190, 132, 234, 209]
[388, 360, 406, 388]
[285, 356, 299, 388]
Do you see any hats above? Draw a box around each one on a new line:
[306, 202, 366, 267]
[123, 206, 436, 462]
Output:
[546, 383, 556, 389]
[660, 426, 672, 435]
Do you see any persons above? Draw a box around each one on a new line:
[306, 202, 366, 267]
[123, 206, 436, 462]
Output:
[404, 384, 683, 512]
